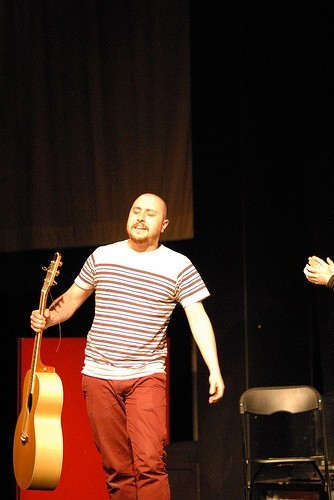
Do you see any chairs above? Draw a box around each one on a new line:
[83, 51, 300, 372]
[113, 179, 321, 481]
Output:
[239, 385, 334, 500]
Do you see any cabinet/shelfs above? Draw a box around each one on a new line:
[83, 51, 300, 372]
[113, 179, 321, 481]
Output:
[17, 336, 111, 500]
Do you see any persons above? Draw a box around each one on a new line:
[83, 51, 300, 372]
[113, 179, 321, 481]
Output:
[30, 190, 224, 500]
[304, 254, 334, 289]
